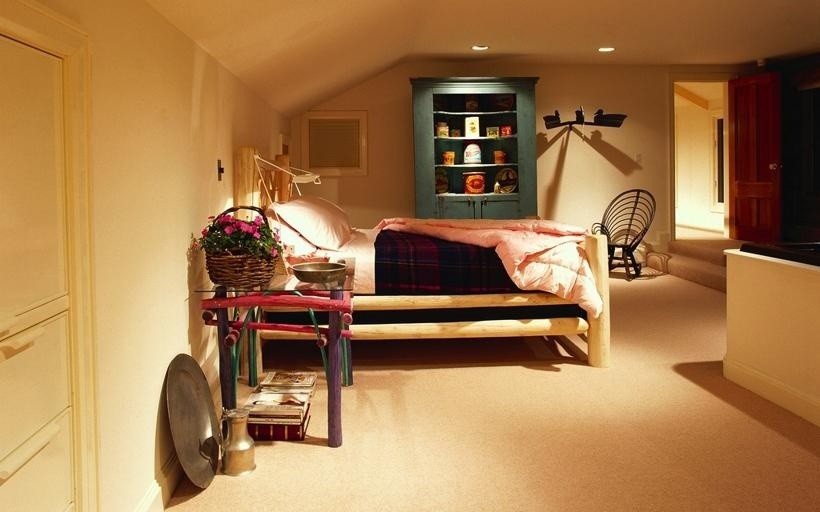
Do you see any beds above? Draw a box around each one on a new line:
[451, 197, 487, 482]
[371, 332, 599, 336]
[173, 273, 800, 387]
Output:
[247, 200, 611, 374]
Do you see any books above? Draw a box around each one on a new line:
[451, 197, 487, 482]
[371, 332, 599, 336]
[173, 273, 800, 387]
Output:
[241, 371, 318, 441]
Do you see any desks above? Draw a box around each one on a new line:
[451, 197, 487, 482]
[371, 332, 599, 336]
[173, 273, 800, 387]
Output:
[203, 254, 358, 453]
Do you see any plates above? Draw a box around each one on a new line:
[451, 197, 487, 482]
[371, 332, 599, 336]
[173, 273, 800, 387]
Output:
[165, 352, 219, 491]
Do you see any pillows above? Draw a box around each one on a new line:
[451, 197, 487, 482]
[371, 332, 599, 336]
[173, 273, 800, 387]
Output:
[269, 194, 353, 256]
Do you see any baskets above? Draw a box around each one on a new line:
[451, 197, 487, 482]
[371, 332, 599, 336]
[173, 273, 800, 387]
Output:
[205, 206, 278, 288]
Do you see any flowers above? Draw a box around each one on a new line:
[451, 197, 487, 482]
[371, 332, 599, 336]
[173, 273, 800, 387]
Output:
[188, 213, 286, 264]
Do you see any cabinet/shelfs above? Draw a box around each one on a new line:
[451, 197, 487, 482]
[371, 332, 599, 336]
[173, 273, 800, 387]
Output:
[410, 74, 539, 227]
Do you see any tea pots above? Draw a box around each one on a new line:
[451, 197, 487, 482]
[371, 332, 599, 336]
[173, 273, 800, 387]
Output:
[218, 408, 257, 477]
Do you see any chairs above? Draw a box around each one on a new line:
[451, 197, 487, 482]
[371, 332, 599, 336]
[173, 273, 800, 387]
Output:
[593, 188, 658, 283]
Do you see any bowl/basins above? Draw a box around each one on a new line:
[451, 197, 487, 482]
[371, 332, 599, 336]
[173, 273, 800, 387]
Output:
[289, 262, 348, 283]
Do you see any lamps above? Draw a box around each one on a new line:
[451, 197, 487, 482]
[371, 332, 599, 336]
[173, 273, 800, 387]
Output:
[253, 153, 322, 219]
